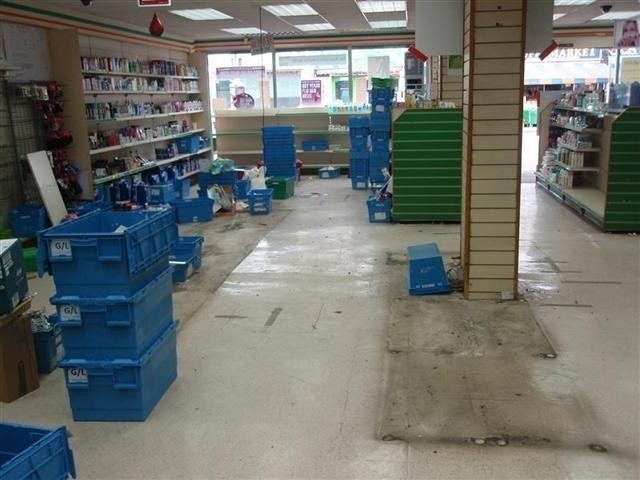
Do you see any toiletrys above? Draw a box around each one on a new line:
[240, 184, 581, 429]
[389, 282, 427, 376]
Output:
[545, 166, 559, 172]
[558, 170, 574, 189]
[560, 147, 585, 167]
[81, 56, 205, 152]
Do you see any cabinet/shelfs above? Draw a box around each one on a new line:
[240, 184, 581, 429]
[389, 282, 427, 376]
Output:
[216, 104, 461, 223]
[48, 27, 214, 201]
[535, 101, 639, 233]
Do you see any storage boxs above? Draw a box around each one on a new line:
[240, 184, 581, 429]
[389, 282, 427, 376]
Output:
[317, 165, 340, 179]
[70, 132, 248, 222]
[405, 242, 449, 295]
[35, 209, 182, 424]
[302, 139, 329, 152]
[171, 237, 202, 280]
[347, 77, 392, 223]
[1, 209, 67, 403]
[2, 421, 77, 480]
[245, 123, 297, 213]
